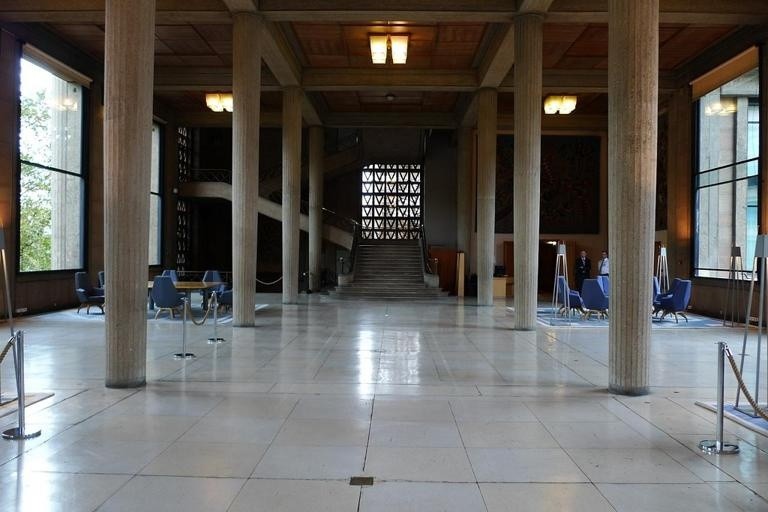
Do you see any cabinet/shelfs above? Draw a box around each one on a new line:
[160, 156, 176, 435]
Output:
[492, 278, 513, 298]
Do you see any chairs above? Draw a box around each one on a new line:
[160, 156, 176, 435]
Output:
[161, 270, 177, 282]
[218, 285, 233, 312]
[200, 270, 221, 312]
[153, 276, 184, 320]
[555, 275, 692, 324]
[98, 270, 104, 293]
[75, 272, 105, 315]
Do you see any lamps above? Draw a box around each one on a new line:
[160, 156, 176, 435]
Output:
[544, 94, 578, 117]
[206, 92, 234, 114]
[366, 32, 410, 63]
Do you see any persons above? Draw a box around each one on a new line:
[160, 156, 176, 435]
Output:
[574, 249, 591, 289]
[597, 250, 609, 276]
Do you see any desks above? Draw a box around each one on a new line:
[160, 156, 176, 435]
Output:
[148, 280, 227, 313]
